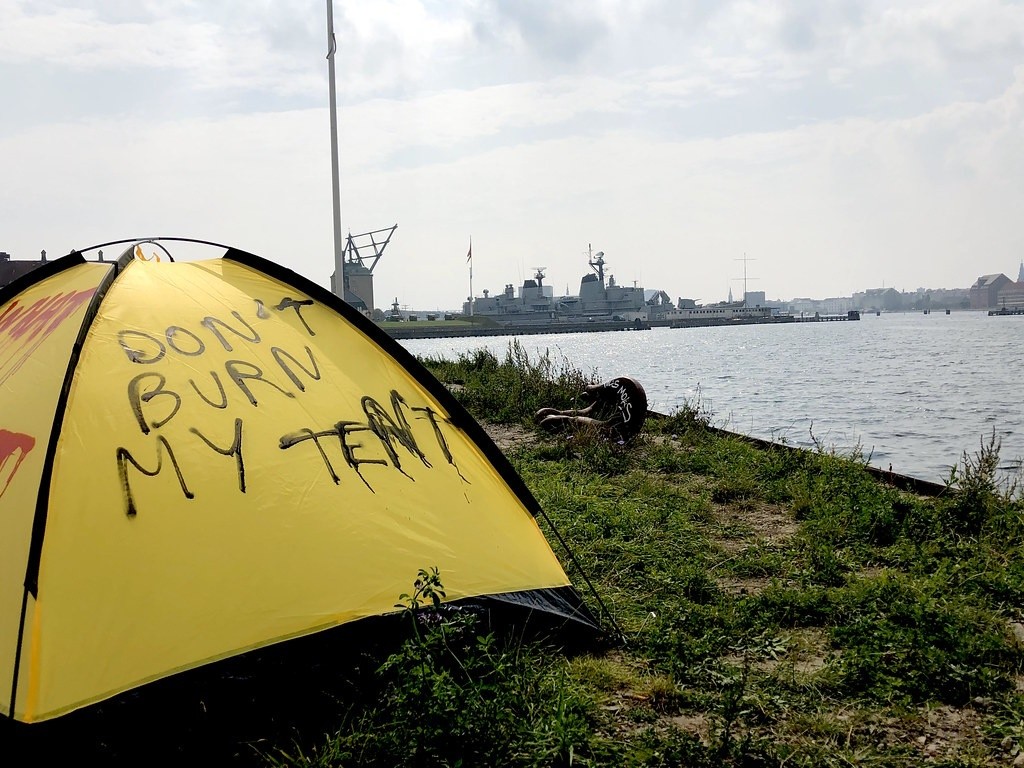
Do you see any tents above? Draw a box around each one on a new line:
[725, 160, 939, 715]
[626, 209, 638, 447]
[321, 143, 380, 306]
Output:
[0, 237, 627, 768]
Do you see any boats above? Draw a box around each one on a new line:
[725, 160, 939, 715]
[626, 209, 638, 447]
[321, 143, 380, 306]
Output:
[556, 244, 644, 313]
[666, 299, 772, 318]
[464, 266, 554, 316]
